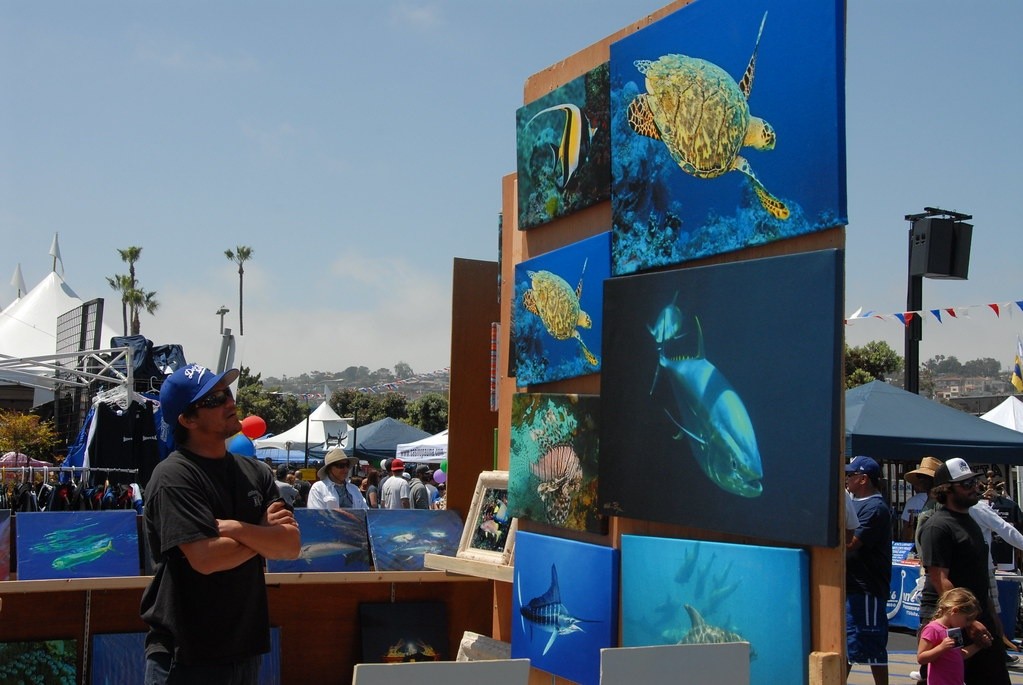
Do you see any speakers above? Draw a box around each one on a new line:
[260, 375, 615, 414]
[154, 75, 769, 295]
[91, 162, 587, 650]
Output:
[909, 219, 974, 281]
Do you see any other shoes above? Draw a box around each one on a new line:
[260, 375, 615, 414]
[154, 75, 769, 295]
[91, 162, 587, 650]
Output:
[1004, 652, 1020, 667]
[910, 670, 924, 682]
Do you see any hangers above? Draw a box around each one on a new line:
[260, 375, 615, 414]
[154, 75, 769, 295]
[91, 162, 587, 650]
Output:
[1, 466, 137, 496]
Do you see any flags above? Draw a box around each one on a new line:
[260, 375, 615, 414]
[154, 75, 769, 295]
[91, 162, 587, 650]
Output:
[1011, 337, 1023, 391]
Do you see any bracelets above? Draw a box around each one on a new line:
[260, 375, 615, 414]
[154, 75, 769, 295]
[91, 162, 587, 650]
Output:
[907, 524, 913, 528]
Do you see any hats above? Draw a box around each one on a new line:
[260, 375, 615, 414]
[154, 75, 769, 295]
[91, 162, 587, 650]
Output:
[846, 455, 879, 479]
[390, 458, 406, 471]
[159, 363, 240, 428]
[416, 465, 434, 474]
[317, 445, 359, 480]
[903, 456, 945, 492]
[938, 457, 985, 487]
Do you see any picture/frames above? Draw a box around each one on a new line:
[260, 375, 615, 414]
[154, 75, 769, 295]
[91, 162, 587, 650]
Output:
[456, 470, 520, 567]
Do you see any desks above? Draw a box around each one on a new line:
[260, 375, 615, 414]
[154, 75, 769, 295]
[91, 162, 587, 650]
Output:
[887, 565, 1023, 643]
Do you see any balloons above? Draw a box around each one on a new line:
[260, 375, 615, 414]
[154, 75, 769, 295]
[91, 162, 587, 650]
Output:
[434, 470, 446, 483]
[226, 415, 266, 457]
[440, 459, 447, 472]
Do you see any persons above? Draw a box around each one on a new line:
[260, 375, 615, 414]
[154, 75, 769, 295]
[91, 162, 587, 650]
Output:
[901, 457, 1023, 666]
[917, 588, 992, 685]
[844, 488, 861, 544]
[139, 363, 301, 685]
[844, 456, 892, 685]
[262, 449, 446, 509]
[916, 458, 1012, 685]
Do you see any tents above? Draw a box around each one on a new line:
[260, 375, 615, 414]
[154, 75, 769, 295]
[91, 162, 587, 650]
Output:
[250, 433, 324, 464]
[306, 417, 434, 468]
[0, 269, 119, 369]
[252, 401, 354, 466]
[396, 429, 448, 464]
[844, 379, 1023, 510]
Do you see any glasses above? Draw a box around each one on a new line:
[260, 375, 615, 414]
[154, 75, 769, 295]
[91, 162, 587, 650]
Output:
[188, 387, 234, 416]
[916, 473, 928, 481]
[848, 470, 864, 479]
[946, 480, 976, 490]
[330, 462, 350, 470]
